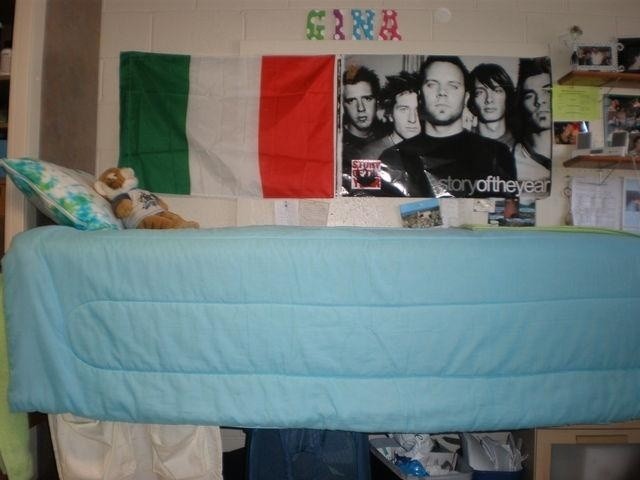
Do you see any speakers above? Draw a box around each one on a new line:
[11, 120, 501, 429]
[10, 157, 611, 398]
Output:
[611, 131, 629, 155]
[576, 132, 592, 149]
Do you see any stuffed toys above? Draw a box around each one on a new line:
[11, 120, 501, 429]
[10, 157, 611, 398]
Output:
[92, 166, 200, 229]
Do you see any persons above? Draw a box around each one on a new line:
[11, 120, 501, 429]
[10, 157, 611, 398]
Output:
[555, 122, 580, 144]
[606, 96, 640, 156]
[342, 55, 551, 198]
[579, 47, 611, 65]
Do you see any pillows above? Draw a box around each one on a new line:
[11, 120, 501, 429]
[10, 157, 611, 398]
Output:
[0, 156, 125, 231]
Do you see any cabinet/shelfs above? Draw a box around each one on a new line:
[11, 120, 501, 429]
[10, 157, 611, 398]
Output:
[514, 422, 640, 480]
[557, 68, 640, 171]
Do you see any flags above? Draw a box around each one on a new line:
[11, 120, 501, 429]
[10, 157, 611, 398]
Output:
[117, 50, 337, 199]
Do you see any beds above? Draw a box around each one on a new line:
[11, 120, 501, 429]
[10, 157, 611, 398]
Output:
[2, 225, 640, 480]
